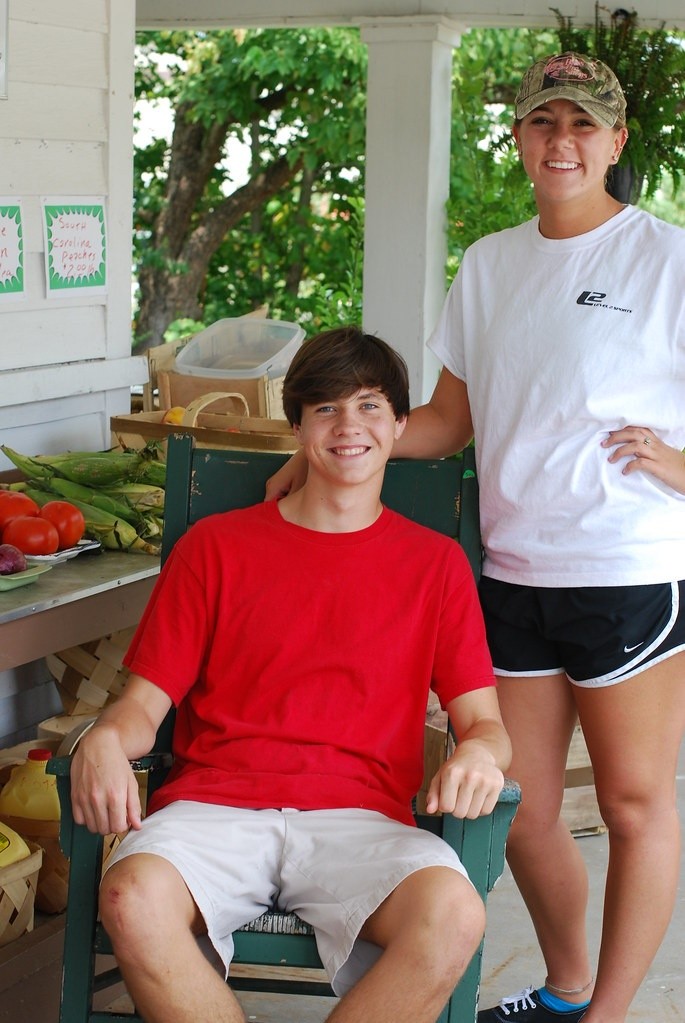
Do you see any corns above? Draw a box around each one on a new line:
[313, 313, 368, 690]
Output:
[0, 444, 167, 555]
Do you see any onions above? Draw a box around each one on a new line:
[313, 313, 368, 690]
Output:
[0, 544, 28, 576]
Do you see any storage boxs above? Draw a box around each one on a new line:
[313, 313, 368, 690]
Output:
[158, 319, 305, 419]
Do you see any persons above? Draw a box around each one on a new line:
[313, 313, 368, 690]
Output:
[263, 54, 685, 1023]
[70, 330, 512, 1023]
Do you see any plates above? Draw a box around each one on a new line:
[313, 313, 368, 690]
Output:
[22, 539, 102, 565]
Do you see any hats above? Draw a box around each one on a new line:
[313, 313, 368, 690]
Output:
[515, 54, 627, 129]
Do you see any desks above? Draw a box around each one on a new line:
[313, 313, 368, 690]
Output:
[0, 547, 162, 674]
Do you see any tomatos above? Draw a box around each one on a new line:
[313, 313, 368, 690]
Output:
[1, 517, 58, 555]
[38, 500, 84, 550]
[0, 491, 41, 537]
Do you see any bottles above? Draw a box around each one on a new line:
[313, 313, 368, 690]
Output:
[0, 749, 61, 821]
[0, 822, 32, 871]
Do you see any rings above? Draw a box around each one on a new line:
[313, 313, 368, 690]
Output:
[645, 437, 651, 445]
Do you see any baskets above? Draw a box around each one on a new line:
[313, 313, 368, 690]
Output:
[0, 836, 44, 946]
[110, 392, 302, 462]
[0, 762, 133, 915]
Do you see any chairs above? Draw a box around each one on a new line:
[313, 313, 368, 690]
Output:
[45, 444, 521, 1023]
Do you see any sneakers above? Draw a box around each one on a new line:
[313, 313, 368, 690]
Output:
[477, 985, 589, 1023]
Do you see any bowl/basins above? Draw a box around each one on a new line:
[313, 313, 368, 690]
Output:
[172, 318, 306, 380]
